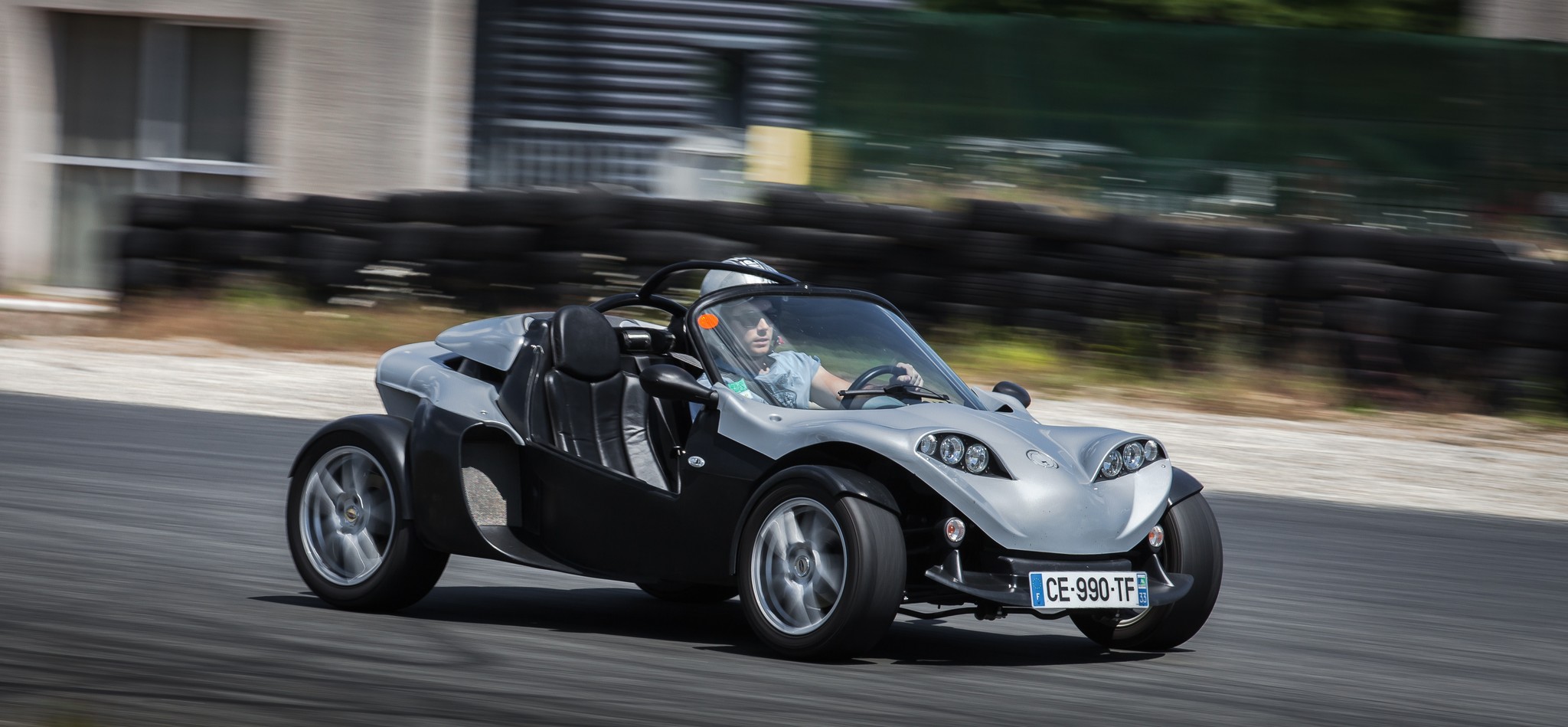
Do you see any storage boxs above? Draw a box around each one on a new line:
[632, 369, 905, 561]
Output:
[745, 125, 850, 188]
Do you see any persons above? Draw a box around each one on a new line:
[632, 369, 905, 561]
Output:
[688, 257, 924, 424]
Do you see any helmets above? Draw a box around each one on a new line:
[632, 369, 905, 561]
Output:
[699, 257, 788, 336]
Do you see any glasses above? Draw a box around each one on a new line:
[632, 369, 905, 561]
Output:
[727, 309, 779, 327]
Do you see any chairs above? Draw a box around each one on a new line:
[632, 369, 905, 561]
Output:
[544, 305, 671, 491]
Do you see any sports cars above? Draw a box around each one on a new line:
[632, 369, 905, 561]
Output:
[288, 260, 1225, 661]
